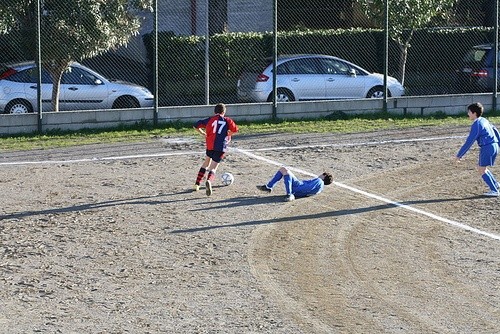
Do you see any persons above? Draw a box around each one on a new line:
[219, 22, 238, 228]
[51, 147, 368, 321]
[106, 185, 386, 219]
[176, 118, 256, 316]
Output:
[256, 167, 333, 201]
[454, 103, 500, 196]
[193, 103, 239, 196]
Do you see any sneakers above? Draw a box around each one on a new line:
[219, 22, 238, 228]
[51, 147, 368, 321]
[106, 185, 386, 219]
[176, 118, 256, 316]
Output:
[256, 185, 271, 193]
[205, 180, 211, 196]
[283, 194, 296, 202]
[193, 185, 199, 192]
[482, 188, 500, 196]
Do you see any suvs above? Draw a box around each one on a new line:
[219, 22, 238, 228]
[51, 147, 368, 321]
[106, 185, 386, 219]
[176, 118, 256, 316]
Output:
[236, 53, 406, 102]
[450, 44, 500, 93]
[0, 58, 155, 114]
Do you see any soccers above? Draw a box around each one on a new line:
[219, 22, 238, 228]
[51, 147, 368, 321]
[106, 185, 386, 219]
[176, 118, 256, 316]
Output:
[219, 172, 234, 186]
